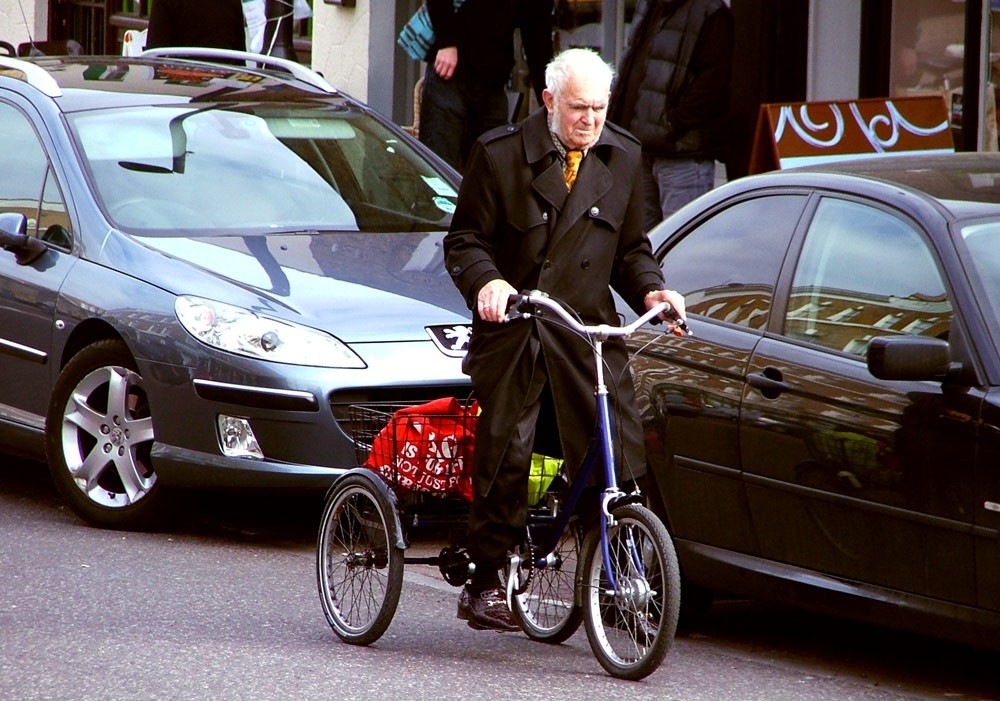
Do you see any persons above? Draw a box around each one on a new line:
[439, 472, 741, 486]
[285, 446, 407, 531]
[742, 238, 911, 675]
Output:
[417, 0, 555, 178]
[444, 49, 687, 634]
[606, 0, 732, 233]
[894, 47, 952, 89]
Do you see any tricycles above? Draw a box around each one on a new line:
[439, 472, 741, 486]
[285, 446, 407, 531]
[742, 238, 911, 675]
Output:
[316, 289, 681, 681]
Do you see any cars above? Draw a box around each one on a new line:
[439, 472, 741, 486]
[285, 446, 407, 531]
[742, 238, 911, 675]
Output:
[609, 153, 1000, 652]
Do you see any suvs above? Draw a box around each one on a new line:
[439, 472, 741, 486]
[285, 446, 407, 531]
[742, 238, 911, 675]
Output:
[0, 47, 475, 530]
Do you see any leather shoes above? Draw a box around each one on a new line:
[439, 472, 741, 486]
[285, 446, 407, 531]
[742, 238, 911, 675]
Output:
[458, 583, 520, 632]
[602, 603, 659, 647]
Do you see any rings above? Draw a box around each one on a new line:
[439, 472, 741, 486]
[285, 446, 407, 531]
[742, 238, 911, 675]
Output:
[483, 304, 490, 309]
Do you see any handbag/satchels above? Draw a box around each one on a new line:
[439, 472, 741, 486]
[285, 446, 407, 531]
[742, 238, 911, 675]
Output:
[397, 0, 464, 60]
[364, 397, 480, 501]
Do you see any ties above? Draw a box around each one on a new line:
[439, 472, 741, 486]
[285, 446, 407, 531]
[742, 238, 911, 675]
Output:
[562, 151, 583, 193]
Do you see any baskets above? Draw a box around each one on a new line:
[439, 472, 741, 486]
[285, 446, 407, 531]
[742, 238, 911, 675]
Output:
[348, 401, 480, 514]
